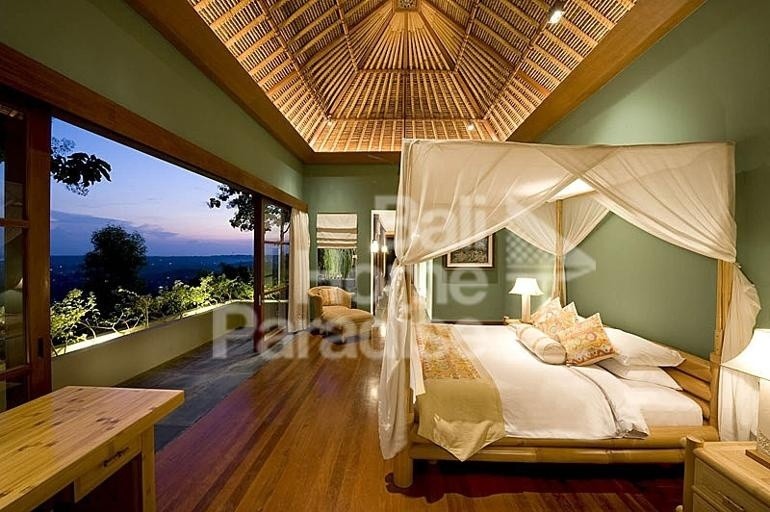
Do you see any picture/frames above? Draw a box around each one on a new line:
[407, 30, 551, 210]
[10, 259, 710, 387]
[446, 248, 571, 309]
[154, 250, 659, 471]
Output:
[443, 233, 495, 271]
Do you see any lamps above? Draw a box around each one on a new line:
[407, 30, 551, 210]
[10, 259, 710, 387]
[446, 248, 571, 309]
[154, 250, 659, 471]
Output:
[719, 328, 770, 470]
[508, 278, 545, 322]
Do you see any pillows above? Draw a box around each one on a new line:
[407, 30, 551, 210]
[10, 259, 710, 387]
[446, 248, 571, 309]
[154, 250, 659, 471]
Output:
[515, 296, 621, 366]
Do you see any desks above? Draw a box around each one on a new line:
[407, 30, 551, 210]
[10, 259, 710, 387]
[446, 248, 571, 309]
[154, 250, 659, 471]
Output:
[0, 386, 185, 512]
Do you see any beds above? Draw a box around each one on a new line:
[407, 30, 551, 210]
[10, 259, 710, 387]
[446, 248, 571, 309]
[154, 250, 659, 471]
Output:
[393, 316, 722, 489]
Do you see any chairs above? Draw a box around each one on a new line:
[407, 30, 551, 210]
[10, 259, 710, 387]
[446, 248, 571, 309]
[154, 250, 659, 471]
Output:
[308, 286, 376, 343]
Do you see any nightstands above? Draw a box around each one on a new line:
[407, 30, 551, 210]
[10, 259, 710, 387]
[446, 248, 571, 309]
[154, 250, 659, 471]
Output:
[676, 435, 770, 512]
